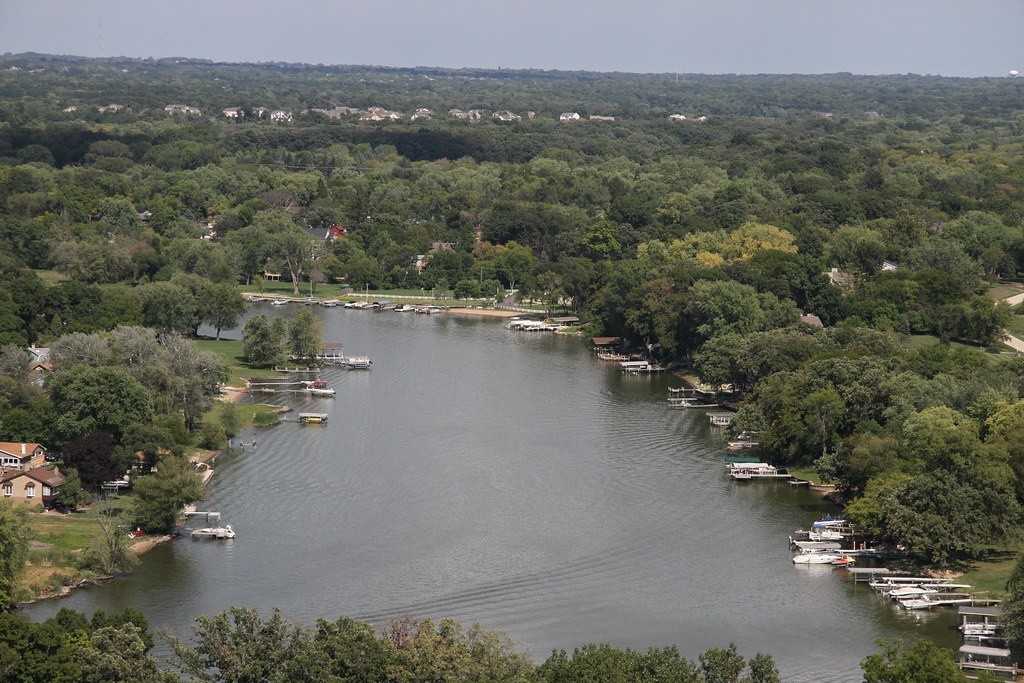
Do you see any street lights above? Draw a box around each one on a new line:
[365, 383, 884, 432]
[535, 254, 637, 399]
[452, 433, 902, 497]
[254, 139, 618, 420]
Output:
[431, 287, 436, 306]
[465, 296, 467, 308]
[365, 283, 370, 303]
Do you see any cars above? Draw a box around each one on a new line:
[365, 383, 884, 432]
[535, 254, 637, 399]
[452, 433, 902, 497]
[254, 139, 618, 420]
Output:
[485, 306, 495, 310]
[467, 306, 474, 309]
[476, 305, 483, 310]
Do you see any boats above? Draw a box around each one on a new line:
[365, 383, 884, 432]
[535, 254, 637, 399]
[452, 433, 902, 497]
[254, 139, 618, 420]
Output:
[306, 418, 323, 423]
[666, 385, 1024, 683]
[245, 296, 449, 314]
[340, 358, 372, 370]
[191, 527, 236, 540]
[503, 316, 668, 373]
[306, 381, 327, 389]
